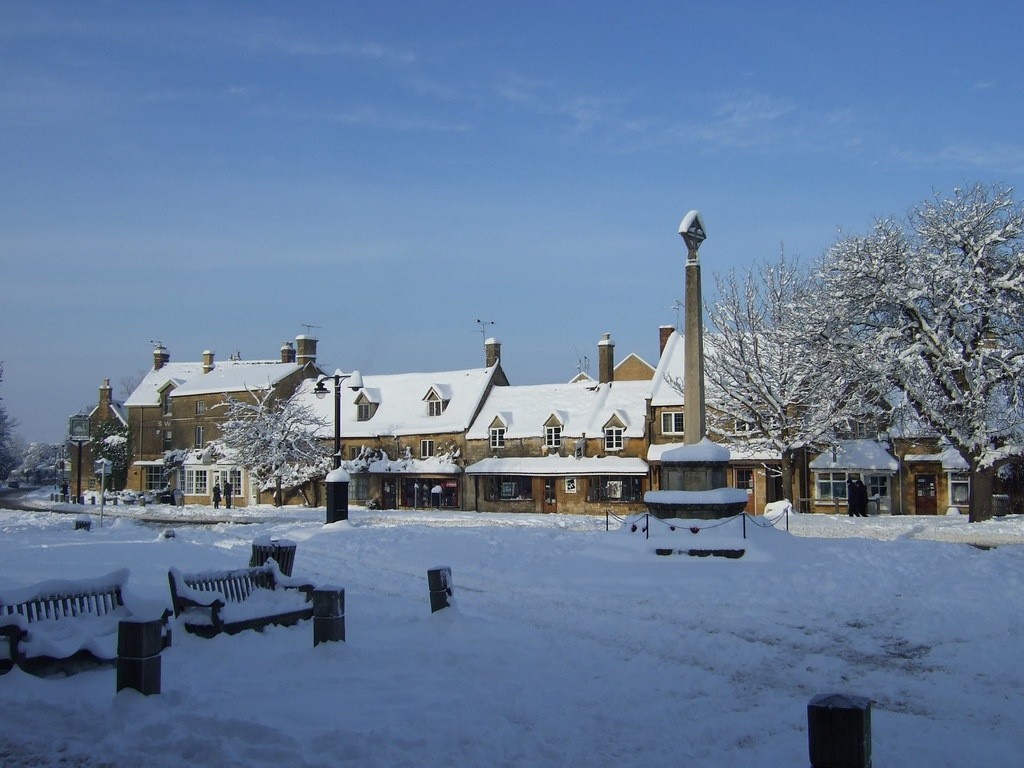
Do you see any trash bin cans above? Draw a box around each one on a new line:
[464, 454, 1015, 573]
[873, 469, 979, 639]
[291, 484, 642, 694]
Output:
[75, 521, 91, 532]
[993, 494, 1009, 516]
[251, 539, 297, 577]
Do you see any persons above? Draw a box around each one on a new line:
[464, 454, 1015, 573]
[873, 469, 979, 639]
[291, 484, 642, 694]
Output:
[847, 479, 869, 517]
[223, 481, 232, 509]
[213, 484, 221, 509]
[63, 482, 68, 494]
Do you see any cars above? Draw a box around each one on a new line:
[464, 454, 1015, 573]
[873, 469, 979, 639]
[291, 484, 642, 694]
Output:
[8, 481, 18, 488]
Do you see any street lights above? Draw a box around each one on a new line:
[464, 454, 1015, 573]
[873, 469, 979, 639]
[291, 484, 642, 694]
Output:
[95, 458, 112, 526]
[312, 372, 364, 524]
